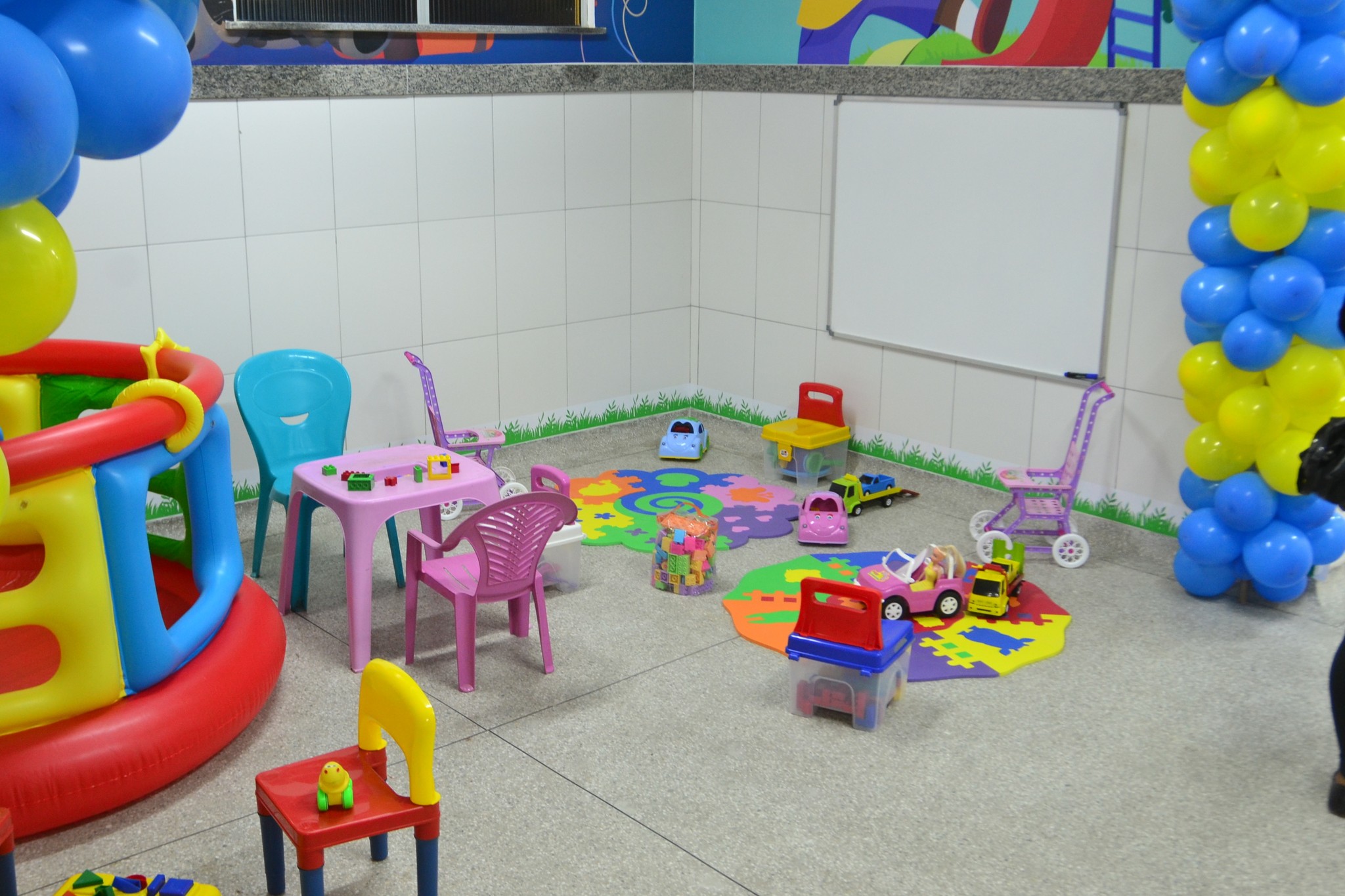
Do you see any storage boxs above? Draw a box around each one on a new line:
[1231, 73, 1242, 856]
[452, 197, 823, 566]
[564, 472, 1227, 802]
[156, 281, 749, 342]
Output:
[527, 463, 586, 601]
[761, 381, 850, 490]
[787, 576, 917, 732]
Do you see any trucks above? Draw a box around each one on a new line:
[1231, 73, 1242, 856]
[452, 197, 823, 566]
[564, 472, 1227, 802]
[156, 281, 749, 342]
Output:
[968, 563, 1024, 618]
[858, 472, 896, 496]
[990, 555, 1020, 586]
[827, 470, 919, 516]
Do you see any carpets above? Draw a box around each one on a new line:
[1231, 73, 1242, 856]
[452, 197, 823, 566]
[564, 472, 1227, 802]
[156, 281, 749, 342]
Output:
[719, 551, 1072, 682]
[555, 468, 803, 555]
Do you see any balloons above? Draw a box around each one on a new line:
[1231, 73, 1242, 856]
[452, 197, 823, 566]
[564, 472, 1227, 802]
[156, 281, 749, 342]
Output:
[1168, 0, 1344, 608]
[0, 0, 200, 357]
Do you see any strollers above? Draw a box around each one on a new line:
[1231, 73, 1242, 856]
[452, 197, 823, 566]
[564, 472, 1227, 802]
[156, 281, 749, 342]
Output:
[969, 380, 1114, 572]
[402, 347, 533, 521]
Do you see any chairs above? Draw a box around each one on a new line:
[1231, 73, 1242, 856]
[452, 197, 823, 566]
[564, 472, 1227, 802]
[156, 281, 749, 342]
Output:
[233, 348, 408, 614]
[404, 492, 580, 692]
[253, 655, 440, 896]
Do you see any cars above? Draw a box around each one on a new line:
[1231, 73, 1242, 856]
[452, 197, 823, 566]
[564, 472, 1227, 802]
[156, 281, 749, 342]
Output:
[796, 492, 848, 546]
[854, 544, 964, 621]
[657, 417, 711, 462]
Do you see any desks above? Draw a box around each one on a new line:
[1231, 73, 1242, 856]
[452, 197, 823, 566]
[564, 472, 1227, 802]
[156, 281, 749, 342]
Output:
[275, 441, 516, 673]
